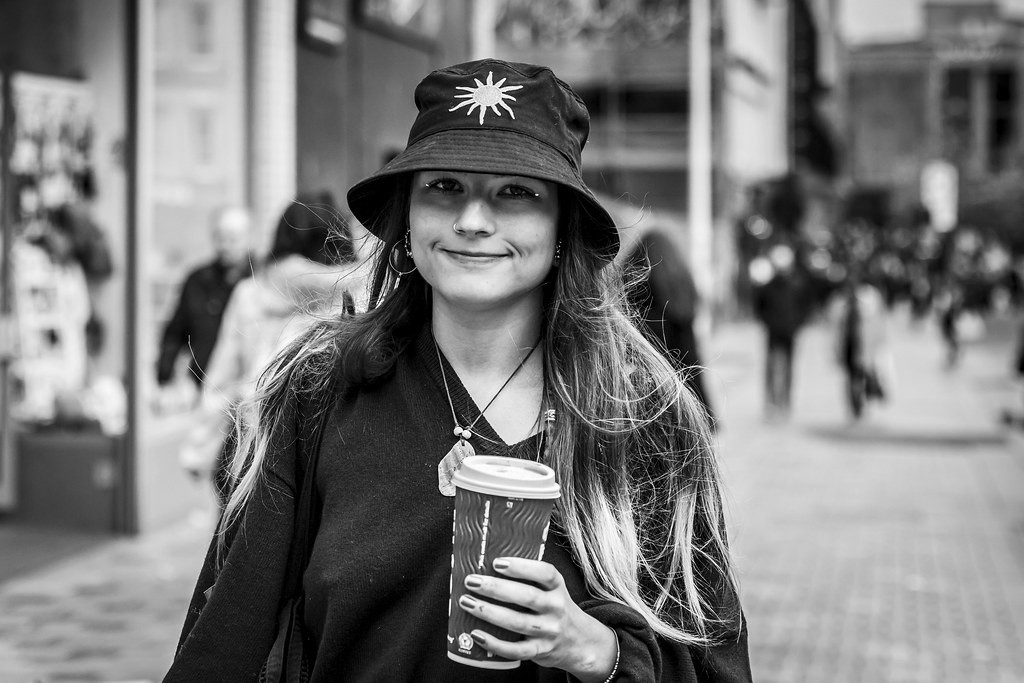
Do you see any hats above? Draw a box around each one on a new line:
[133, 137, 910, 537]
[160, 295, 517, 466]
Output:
[346, 59, 620, 271]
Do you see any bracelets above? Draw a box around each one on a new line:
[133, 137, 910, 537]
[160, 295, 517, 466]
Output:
[604, 626, 620, 683]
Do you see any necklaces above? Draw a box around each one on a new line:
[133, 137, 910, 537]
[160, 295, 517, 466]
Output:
[430, 326, 543, 497]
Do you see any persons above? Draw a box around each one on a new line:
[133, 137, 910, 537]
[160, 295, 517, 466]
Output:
[12, 169, 111, 366]
[155, 189, 372, 506]
[621, 202, 1024, 432]
[161, 59, 752, 683]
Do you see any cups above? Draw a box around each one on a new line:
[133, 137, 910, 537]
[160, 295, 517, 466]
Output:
[447, 456, 562, 670]
